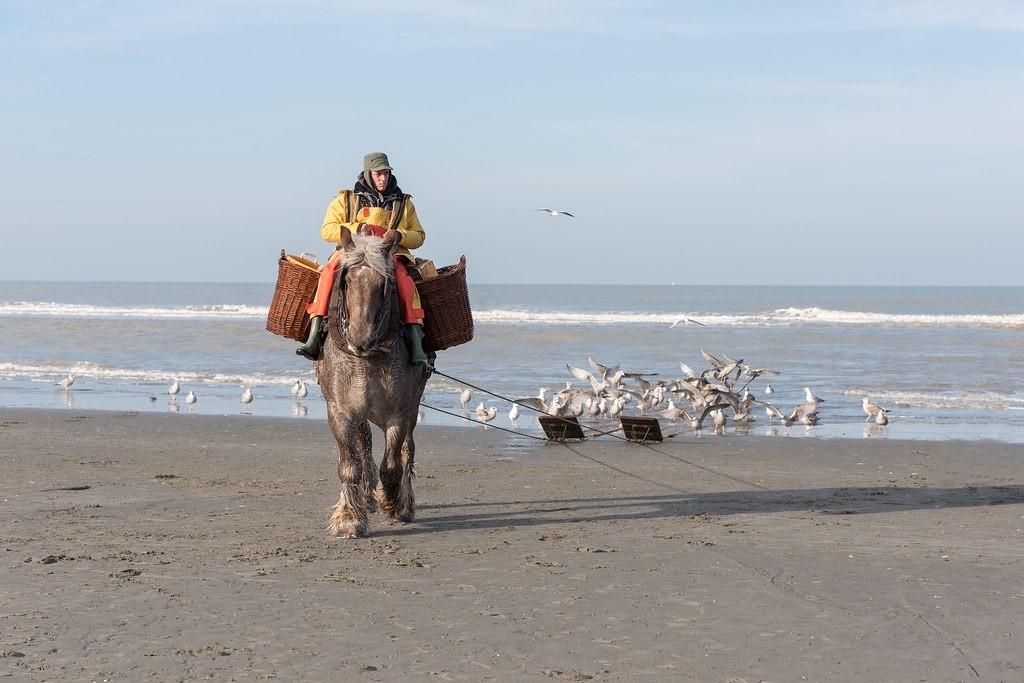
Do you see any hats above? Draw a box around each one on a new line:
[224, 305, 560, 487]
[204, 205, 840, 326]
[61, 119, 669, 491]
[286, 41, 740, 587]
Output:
[363, 153, 394, 191]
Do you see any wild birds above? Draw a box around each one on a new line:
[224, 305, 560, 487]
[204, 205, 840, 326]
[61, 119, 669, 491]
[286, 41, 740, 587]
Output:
[528, 205, 574, 221]
[456, 384, 501, 431]
[507, 317, 829, 437]
[56, 373, 75, 390]
[290, 378, 308, 400]
[186, 391, 197, 411]
[168, 381, 181, 401]
[860, 396, 894, 432]
[239, 386, 253, 410]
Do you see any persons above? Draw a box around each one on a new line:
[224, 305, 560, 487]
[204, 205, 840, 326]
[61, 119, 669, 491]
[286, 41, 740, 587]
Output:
[294, 151, 430, 364]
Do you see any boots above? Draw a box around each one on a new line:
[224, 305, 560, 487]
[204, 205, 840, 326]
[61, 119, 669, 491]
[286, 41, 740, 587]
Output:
[296, 317, 324, 361]
[404, 325, 428, 365]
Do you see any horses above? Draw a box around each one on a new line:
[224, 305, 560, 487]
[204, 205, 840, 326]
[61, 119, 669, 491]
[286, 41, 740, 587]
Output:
[309, 224, 432, 537]
